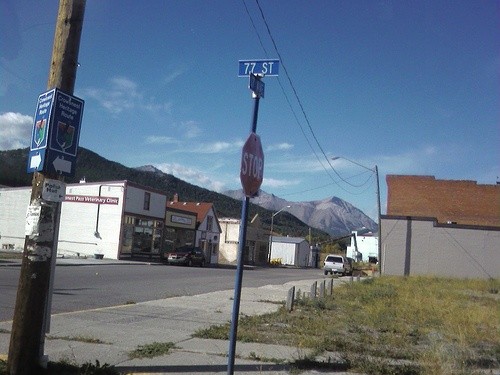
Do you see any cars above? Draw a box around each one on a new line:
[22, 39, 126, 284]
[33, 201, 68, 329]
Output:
[167, 246, 206, 267]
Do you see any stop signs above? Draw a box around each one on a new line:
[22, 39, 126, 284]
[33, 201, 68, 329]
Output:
[240, 131, 265, 197]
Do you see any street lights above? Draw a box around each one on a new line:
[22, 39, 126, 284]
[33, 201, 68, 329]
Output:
[332, 156, 381, 275]
[266, 206, 291, 265]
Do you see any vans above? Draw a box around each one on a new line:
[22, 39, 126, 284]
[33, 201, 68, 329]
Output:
[324, 255, 354, 276]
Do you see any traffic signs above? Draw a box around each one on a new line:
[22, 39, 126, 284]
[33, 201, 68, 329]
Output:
[27, 89, 86, 176]
